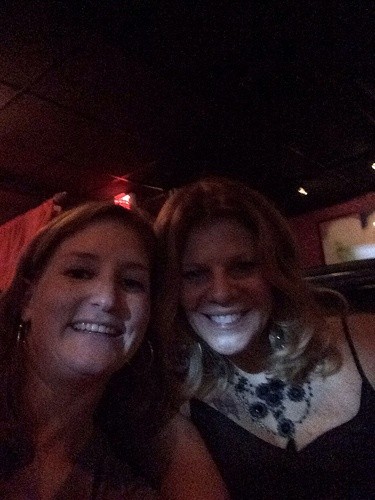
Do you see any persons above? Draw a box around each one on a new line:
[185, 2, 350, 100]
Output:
[110, 176, 374, 500]
[0, 202, 158, 500]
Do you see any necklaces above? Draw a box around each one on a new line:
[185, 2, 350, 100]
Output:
[228, 358, 313, 456]
